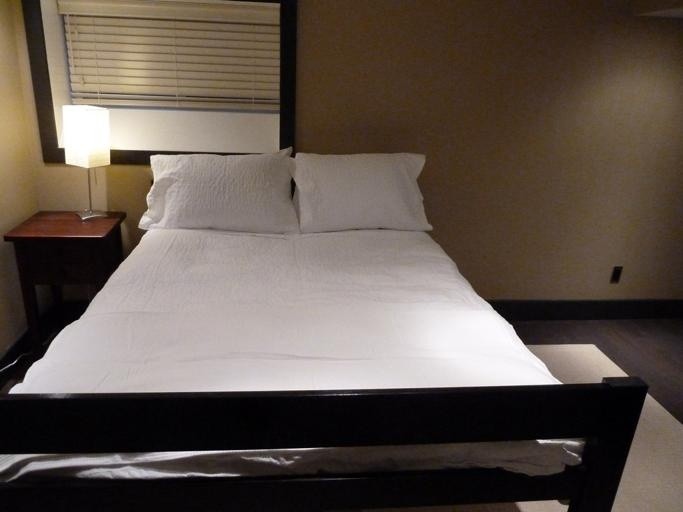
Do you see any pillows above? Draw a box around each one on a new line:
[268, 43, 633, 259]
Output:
[140, 146, 299, 235]
[291, 151, 435, 231]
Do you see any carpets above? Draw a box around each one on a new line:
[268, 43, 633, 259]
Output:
[513, 343, 682, 511]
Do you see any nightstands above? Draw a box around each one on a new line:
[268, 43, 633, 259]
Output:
[2, 210, 126, 333]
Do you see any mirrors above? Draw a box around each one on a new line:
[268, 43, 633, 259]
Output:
[23, 0, 296, 157]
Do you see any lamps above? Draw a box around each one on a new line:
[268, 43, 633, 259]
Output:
[59, 102, 112, 221]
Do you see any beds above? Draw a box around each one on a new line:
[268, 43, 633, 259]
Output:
[1, 177, 649, 512]
